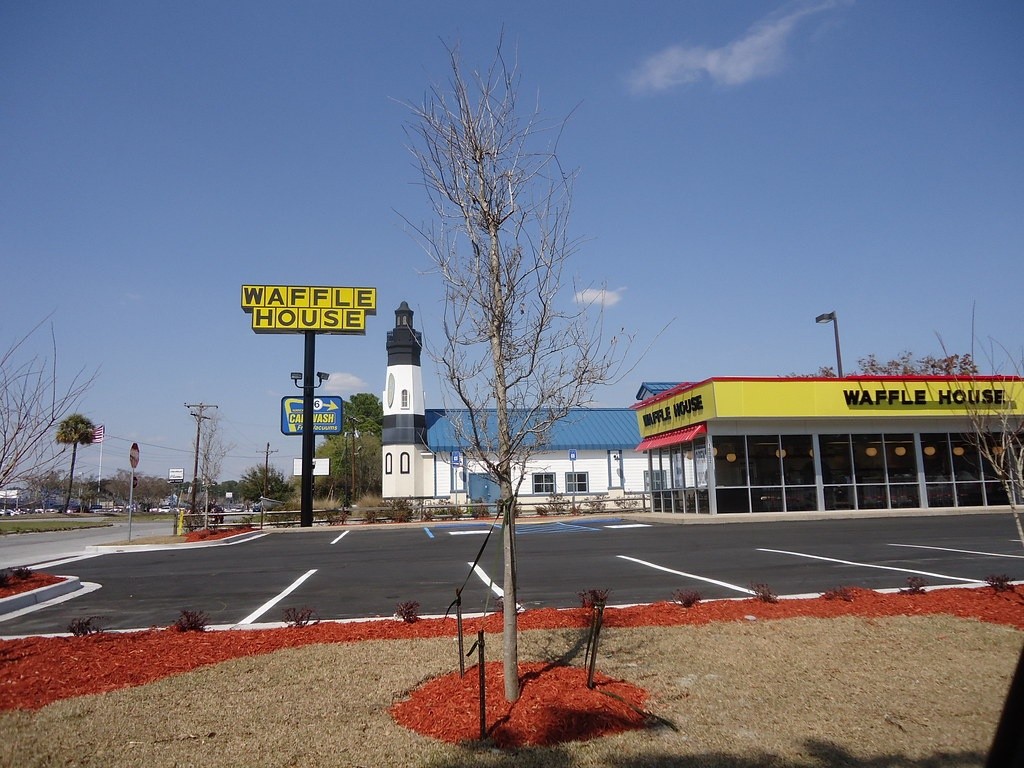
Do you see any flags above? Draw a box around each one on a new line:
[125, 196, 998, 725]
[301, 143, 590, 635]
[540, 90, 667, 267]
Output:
[92, 426, 103, 442]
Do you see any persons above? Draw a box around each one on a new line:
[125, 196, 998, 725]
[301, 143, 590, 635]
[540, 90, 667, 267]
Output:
[215, 505, 224, 524]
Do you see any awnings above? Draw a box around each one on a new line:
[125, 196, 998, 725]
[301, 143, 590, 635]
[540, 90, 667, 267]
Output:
[636, 425, 706, 451]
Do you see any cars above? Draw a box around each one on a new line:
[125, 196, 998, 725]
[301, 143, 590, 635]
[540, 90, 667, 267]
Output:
[231, 506, 243, 512]
[253, 506, 260, 512]
[0, 508, 59, 516]
[150, 506, 180, 513]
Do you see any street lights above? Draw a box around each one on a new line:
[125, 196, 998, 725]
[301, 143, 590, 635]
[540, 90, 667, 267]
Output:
[816, 311, 843, 377]
